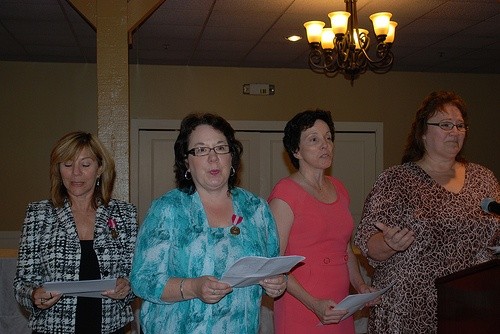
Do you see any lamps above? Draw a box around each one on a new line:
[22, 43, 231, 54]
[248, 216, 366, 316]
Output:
[303, 0, 398, 87]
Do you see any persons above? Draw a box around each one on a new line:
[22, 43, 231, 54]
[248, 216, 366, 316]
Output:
[353, 91, 500, 334]
[12, 131, 138, 334]
[131, 112, 288, 334]
[267, 109, 381, 334]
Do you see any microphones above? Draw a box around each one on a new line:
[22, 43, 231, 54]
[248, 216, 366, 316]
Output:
[480, 198, 500, 214]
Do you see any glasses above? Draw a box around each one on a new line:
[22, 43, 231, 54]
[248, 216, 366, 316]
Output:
[186, 145, 234, 156]
[425, 121, 469, 132]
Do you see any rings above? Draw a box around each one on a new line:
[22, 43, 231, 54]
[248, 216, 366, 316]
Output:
[50, 293, 52, 298]
[41, 298, 45, 304]
[278, 290, 281, 295]
[212, 289, 215, 295]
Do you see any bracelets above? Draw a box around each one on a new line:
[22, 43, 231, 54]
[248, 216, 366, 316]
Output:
[180, 278, 186, 301]
